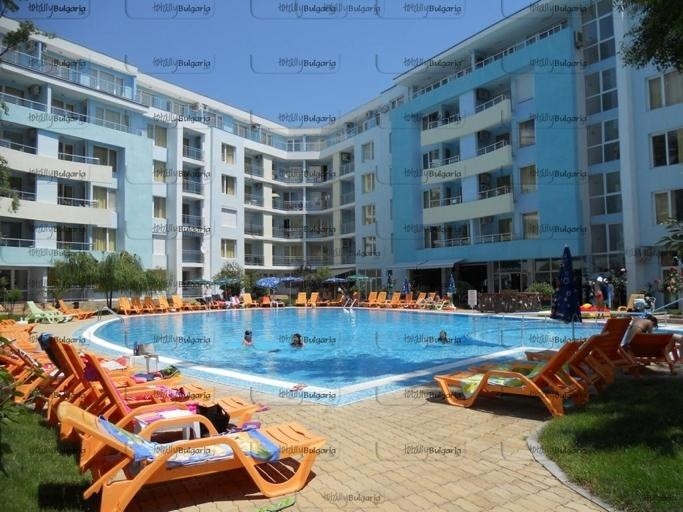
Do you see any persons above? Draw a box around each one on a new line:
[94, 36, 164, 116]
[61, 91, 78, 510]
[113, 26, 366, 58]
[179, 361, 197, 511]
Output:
[623, 313, 658, 349]
[261, 293, 271, 302]
[240, 328, 254, 347]
[592, 286, 606, 319]
[289, 332, 304, 348]
[437, 330, 449, 343]
[231, 294, 239, 308]
[201, 284, 215, 309]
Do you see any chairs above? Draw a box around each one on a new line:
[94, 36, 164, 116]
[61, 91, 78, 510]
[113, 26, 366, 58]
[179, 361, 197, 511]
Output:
[24, 287, 454, 324]
[0, 316, 324, 511]
[436, 315, 677, 419]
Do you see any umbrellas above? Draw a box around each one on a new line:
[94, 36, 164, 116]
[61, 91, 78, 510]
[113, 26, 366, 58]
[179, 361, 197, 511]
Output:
[400, 277, 410, 295]
[217, 275, 242, 300]
[446, 274, 458, 305]
[282, 273, 304, 303]
[256, 274, 282, 300]
[347, 272, 369, 298]
[323, 274, 344, 298]
[551, 244, 583, 340]
